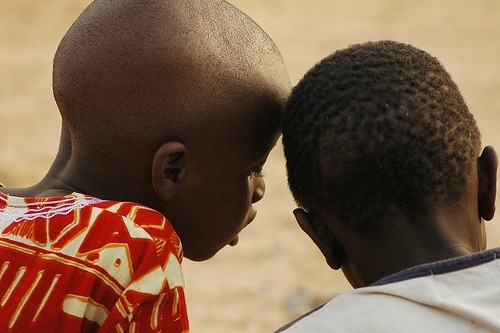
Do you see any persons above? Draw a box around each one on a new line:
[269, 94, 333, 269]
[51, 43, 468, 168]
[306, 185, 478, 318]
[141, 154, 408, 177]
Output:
[0, 0, 292, 332]
[272, 41, 500, 332]
